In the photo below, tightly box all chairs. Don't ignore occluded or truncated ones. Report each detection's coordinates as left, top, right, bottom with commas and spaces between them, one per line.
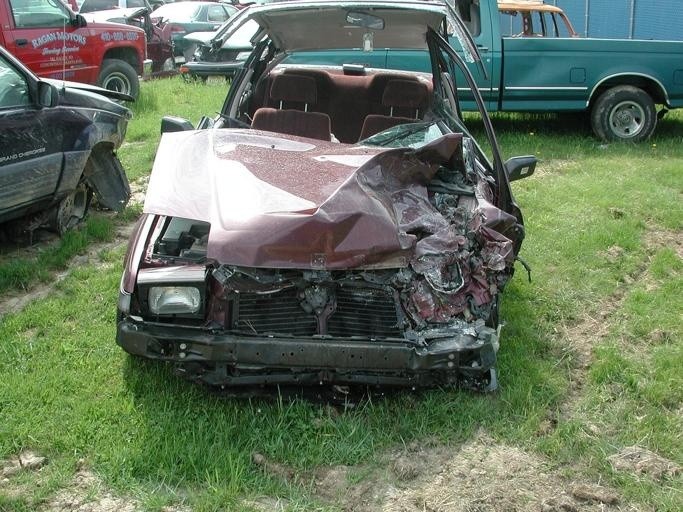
248, 62, 435, 143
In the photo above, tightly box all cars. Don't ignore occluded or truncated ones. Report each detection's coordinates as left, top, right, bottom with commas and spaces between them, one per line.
0, 43, 136, 244
79, 0, 683, 145
113, 2, 538, 398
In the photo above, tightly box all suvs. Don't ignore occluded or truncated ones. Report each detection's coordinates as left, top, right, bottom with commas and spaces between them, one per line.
0, 0, 147, 106
